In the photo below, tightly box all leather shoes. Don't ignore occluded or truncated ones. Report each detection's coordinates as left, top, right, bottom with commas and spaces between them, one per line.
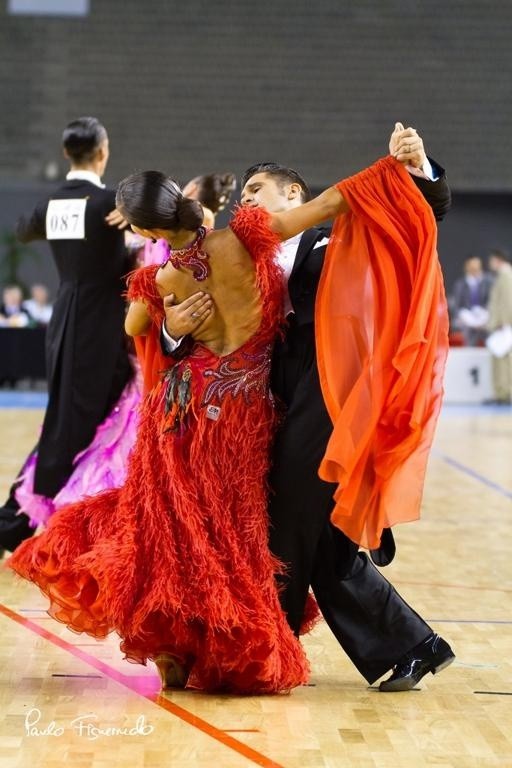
379, 633, 456, 692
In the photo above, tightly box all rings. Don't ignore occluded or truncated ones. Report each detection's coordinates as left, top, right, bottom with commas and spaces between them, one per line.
192, 312, 200, 319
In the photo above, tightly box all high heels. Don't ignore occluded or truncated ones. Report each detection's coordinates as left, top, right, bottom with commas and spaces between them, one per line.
154, 654, 189, 689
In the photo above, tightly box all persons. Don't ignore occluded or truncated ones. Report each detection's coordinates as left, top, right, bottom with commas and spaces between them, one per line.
23, 282, 53, 388
160, 122, 456, 692
446, 255, 494, 347
14, 173, 237, 529
0, 286, 32, 389
2, 126, 410, 695
0, 116, 157, 560
474, 249, 512, 405
